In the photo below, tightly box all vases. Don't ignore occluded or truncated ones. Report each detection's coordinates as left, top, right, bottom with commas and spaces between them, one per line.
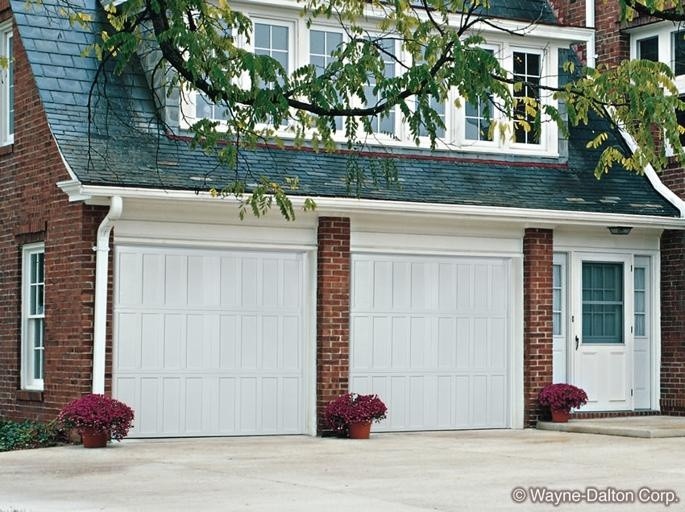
550, 406, 570, 422
348, 421, 371, 439
82, 428, 106, 448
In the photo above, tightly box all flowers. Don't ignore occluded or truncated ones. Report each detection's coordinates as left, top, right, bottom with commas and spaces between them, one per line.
538, 384, 586, 412
56, 393, 135, 441
324, 393, 387, 433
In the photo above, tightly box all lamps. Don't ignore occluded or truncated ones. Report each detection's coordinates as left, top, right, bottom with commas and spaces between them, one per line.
608, 227, 632, 234
